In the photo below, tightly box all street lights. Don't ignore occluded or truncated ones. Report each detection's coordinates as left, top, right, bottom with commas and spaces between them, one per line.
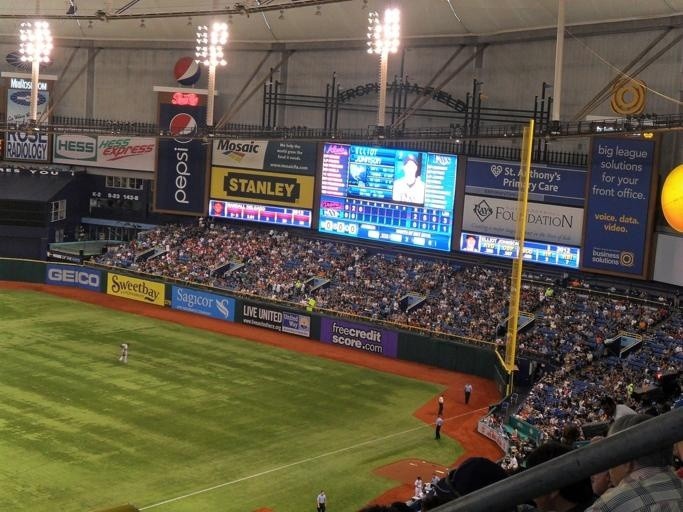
19, 20, 54, 127
365, 7, 403, 138
195, 22, 229, 127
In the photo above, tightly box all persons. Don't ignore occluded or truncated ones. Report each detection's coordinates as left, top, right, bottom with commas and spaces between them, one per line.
212, 201, 225, 216
392, 156, 425, 204
